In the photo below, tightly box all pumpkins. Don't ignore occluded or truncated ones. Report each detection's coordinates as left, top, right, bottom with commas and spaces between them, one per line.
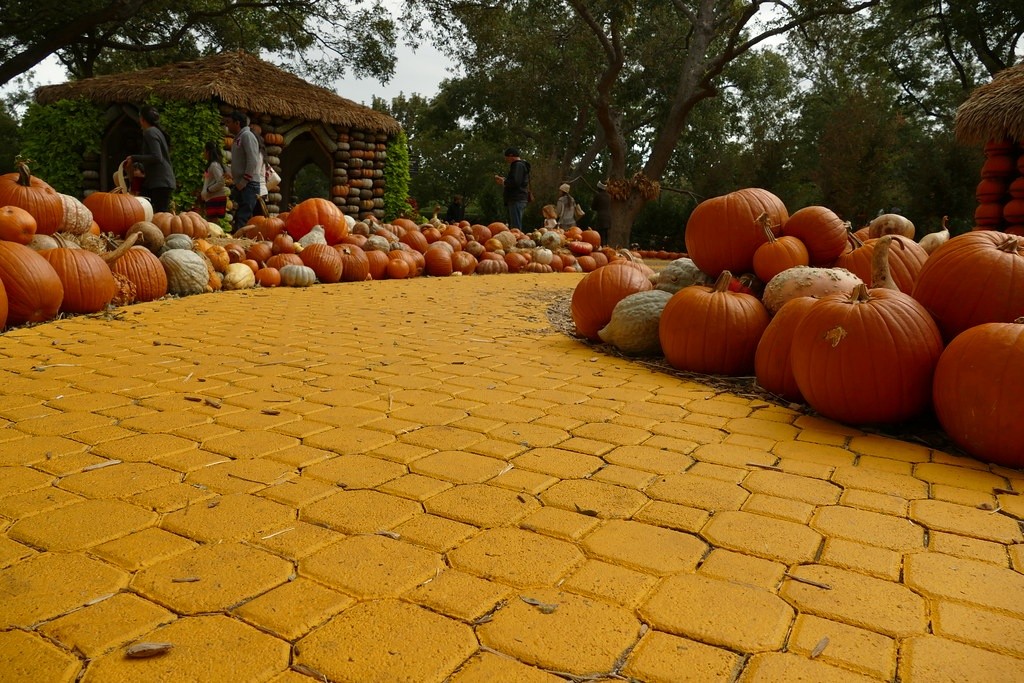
0, 105, 609, 332
569, 137, 1024, 469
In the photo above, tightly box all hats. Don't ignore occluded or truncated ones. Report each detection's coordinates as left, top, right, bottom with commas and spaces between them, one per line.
113, 160, 134, 196
504, 148, 520, 157
558, 183, 570, 194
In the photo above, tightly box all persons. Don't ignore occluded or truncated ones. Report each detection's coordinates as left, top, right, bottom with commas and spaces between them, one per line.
542, 205, 559, 230
446, 195, 461, 224
226, 111, 260, 235
494, 148, 532, 231
249, 125, 269, 218
199, 140, 226, 226
552, 184, 576, 230
125, 110, 176, 215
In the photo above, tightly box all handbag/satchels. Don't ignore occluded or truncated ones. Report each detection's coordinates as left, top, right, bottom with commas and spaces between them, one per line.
526, 191, 535, 205
574, 204, 585, 221
264, 160, 281, 192
